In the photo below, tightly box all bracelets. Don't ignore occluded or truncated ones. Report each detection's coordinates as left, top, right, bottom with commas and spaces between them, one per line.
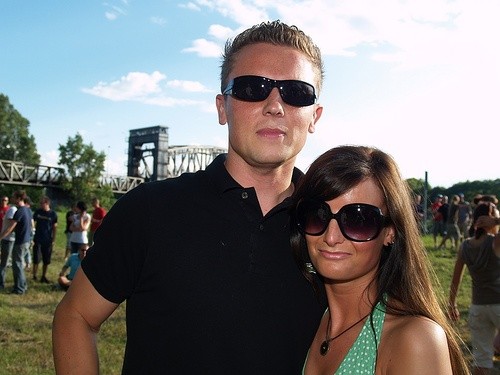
449, 304, 457, 309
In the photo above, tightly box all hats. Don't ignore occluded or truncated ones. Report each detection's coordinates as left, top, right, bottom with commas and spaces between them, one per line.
436, 194, 443, 198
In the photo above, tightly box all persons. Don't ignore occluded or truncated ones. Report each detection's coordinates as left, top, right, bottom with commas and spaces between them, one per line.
52, 23, 329, 375
0, 189, 105, 293
413, 193, 500, 375
285, 145, 472, 375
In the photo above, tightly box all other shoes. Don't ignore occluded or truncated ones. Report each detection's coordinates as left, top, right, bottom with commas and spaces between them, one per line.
32, 275, 37, 281
40, 276, 50, 283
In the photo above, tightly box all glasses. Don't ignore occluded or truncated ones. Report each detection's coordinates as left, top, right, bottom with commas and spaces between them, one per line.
223, 75, 317, 107
81, 248, 87, 251
293, 198, 391, 242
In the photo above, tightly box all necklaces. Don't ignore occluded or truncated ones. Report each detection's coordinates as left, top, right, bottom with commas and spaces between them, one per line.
320, 313, 370, 356
